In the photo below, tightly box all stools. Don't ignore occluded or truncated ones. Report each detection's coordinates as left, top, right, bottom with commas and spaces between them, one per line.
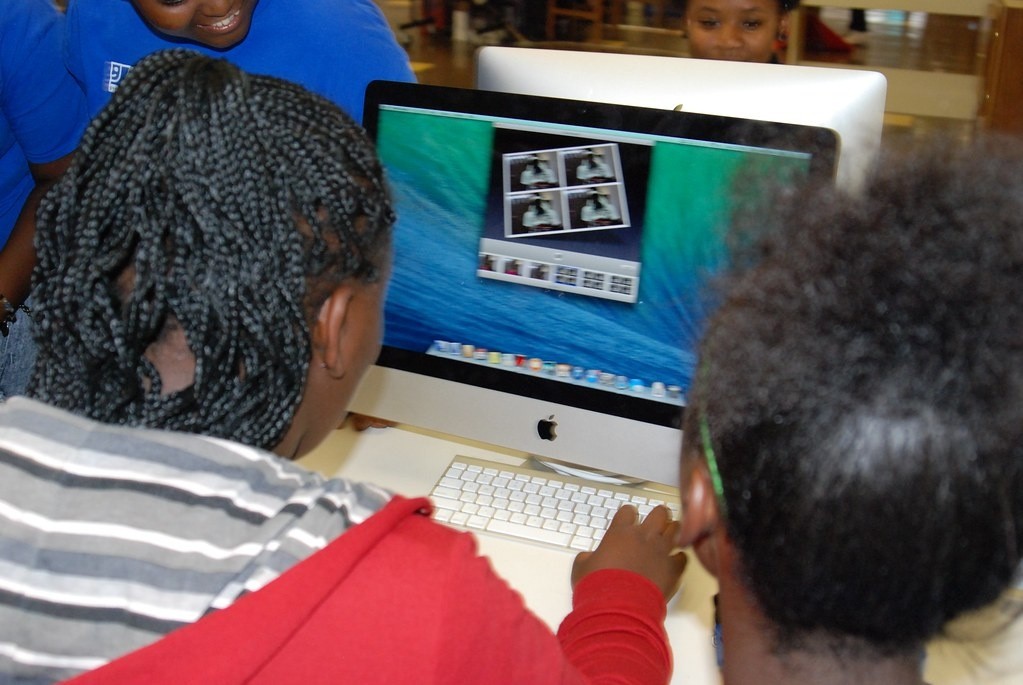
546, 0, 619, 43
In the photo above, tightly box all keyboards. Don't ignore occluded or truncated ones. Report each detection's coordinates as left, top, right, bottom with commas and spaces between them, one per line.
425, 455, 685, 552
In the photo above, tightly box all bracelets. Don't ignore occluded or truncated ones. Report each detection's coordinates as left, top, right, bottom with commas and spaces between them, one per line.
0, 293, 15, 315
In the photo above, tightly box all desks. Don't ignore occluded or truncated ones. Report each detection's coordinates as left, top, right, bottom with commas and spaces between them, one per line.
292, 417, 1023, 684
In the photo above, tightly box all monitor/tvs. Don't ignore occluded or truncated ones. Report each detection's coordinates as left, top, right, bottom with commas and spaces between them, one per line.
346, 46, 887, 492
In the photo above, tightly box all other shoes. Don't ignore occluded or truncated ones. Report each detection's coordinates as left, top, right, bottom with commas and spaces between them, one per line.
842, 29, 871, 47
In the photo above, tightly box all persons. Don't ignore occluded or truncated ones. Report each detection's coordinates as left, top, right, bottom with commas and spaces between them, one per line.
0, 0, 90, 337
0, 49, 688, 685
679, 145, 1023, 685
64, 0, 418, 132
681, 0, 793, 63
